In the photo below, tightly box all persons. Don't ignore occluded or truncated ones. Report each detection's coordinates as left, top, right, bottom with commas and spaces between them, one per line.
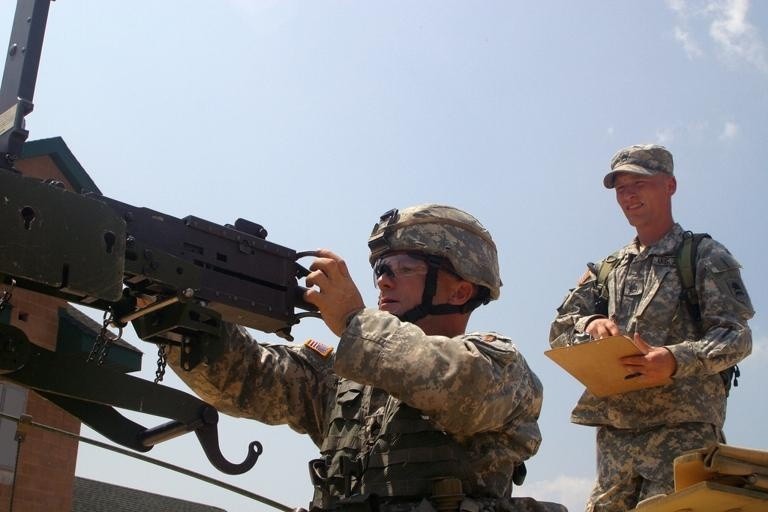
148, 202, 545, 511
546, 143, 756, 511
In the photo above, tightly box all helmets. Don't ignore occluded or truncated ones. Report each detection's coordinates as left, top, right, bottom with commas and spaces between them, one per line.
368, 202, 500, 301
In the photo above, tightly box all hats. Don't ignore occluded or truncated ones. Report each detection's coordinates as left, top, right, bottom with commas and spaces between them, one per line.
602, 144, 674, 189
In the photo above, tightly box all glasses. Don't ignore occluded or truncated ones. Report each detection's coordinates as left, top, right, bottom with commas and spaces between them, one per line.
372, 251, 428, 288
